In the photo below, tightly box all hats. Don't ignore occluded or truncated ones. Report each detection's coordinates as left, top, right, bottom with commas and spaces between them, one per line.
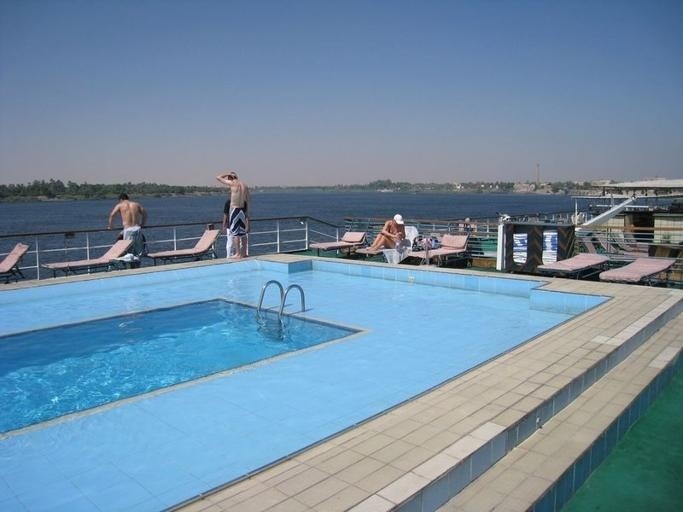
393, 214, 404, 226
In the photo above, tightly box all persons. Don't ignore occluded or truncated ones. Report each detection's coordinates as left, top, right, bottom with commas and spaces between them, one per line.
108, 193, 146, 268
365, 213, 405, 252
222, 198, 248, 257
216, 171, 249, 259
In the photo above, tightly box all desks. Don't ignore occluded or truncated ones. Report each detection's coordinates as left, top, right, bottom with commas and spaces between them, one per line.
110, 258, 140, 269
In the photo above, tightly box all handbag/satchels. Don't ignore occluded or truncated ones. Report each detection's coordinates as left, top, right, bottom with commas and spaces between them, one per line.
411, 235, 440, 253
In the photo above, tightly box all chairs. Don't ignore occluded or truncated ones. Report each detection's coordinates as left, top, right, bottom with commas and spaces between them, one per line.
309, 216, 472, 265
144, 229, 220, 266
40, 239, 135, 277
536, 253, 677, 286
0, 242, 30, 283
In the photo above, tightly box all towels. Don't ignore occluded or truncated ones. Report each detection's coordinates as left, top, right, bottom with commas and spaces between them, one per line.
121, 252, 135, 262
